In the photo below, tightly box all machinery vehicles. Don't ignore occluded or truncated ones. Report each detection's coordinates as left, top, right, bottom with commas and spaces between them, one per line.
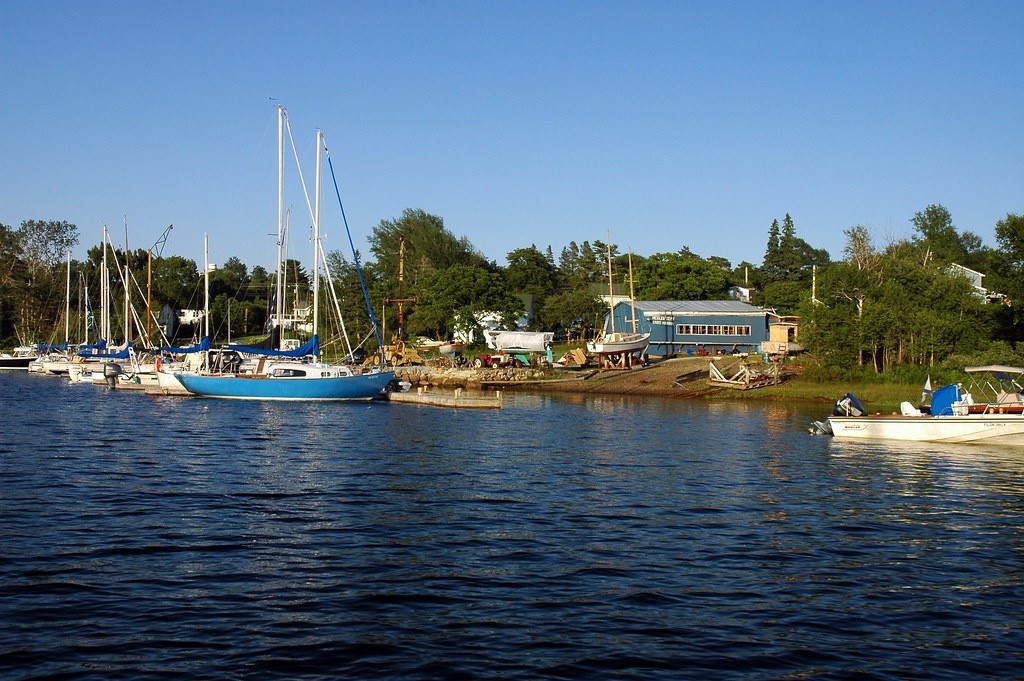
363, 234, 426, 366
474, 353, 521, 369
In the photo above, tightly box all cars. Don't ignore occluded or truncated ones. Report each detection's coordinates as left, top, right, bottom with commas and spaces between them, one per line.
345, 347, 367, 365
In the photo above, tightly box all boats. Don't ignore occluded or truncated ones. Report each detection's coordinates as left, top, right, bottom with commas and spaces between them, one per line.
808, 365, 1024, 440
438, 342, 463, 357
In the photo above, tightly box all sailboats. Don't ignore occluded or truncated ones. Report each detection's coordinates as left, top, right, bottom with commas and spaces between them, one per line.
0, 96, 396, 403
586, 229, 650, 355
